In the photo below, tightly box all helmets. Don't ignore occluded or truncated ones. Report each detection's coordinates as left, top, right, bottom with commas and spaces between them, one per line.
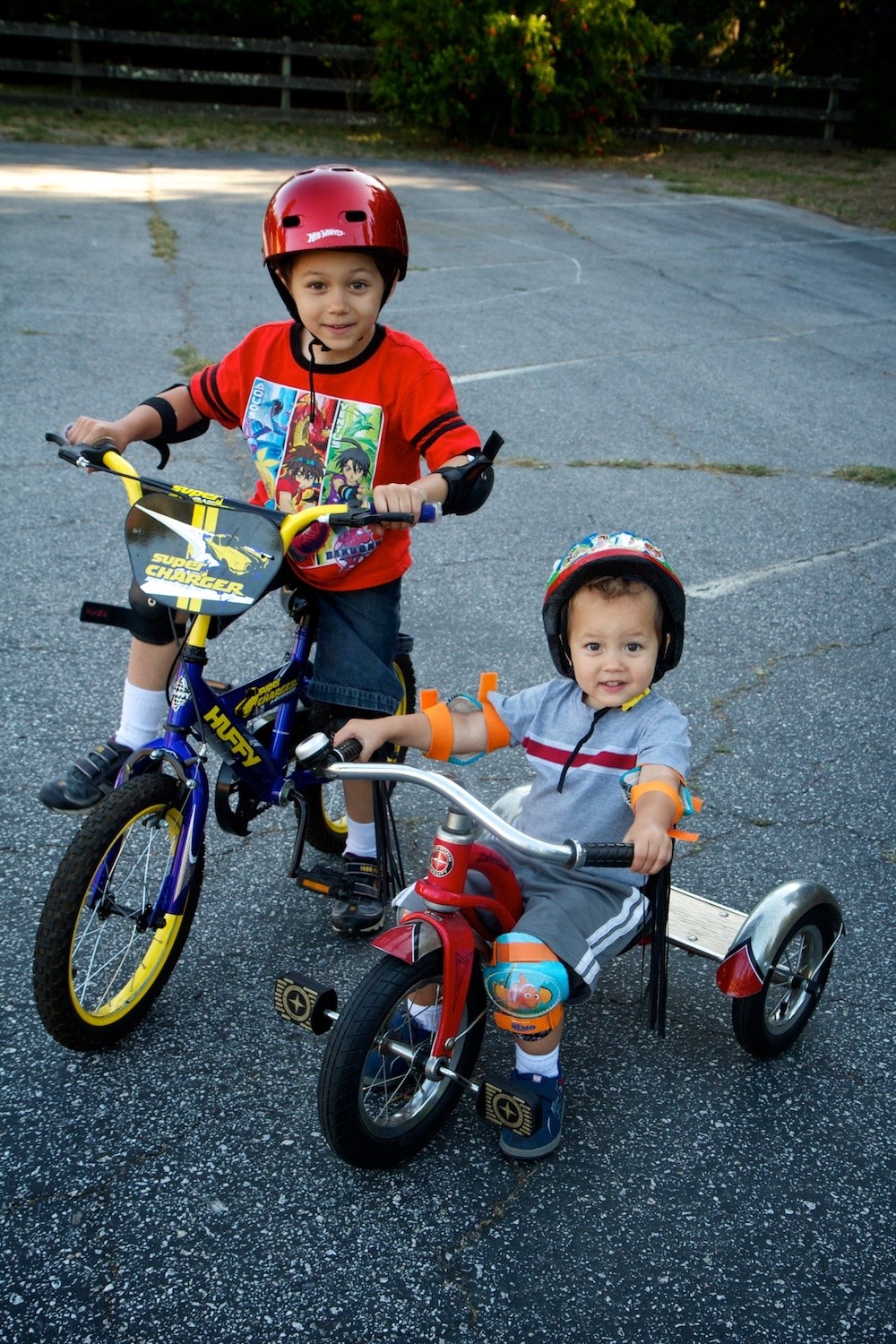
261, 163, 409, 285
540, 532, 686, 677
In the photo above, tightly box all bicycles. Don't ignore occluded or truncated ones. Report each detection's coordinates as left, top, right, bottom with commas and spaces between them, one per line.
32, 424, 438, 1053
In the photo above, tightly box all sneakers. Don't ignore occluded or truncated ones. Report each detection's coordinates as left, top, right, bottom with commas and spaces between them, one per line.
330, 851, 388, 936
500, 1067, 567, 1158
359, 1004, 437, 1088
38, 735, 133, 817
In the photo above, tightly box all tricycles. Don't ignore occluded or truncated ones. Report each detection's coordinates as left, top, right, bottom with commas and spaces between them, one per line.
290, 733, 849, 1166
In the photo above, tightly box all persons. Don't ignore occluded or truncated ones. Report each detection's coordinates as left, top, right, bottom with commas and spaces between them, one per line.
36, 161, 505, 935
333, 532, 703, 1156
